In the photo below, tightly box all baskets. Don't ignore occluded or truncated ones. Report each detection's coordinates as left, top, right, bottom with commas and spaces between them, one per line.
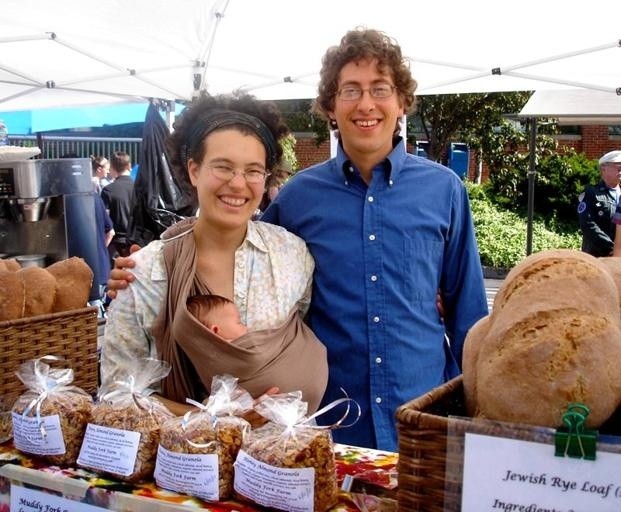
0, 304, 100, 426
394, 373, 621, 512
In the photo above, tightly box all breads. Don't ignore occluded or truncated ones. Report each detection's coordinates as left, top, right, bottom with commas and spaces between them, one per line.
0, 256, 94, 320
462, 248, 621, 430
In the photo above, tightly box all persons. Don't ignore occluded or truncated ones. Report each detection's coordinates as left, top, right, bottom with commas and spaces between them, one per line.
611, 196, 621, 257
97, 87, 449, 433
106, 27, 489, 448
578, 149, 621, 257
260, 161, 294, 212
102, 150, 138, 268
46, 152, 116, 304
184, 293, 249, 343
88, 154, 110, 192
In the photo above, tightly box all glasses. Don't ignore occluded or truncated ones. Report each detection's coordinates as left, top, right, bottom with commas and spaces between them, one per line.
196, 158, 273, 185
336, 83, 398, 101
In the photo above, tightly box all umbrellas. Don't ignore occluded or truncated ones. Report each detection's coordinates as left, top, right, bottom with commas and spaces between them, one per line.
124, 102, 197, 247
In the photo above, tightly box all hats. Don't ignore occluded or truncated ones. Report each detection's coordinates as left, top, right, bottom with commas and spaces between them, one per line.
276, 162, 293, 174
599, 150, 621, 166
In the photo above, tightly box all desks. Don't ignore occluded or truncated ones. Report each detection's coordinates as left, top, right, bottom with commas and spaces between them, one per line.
0, 437, 399, 512
